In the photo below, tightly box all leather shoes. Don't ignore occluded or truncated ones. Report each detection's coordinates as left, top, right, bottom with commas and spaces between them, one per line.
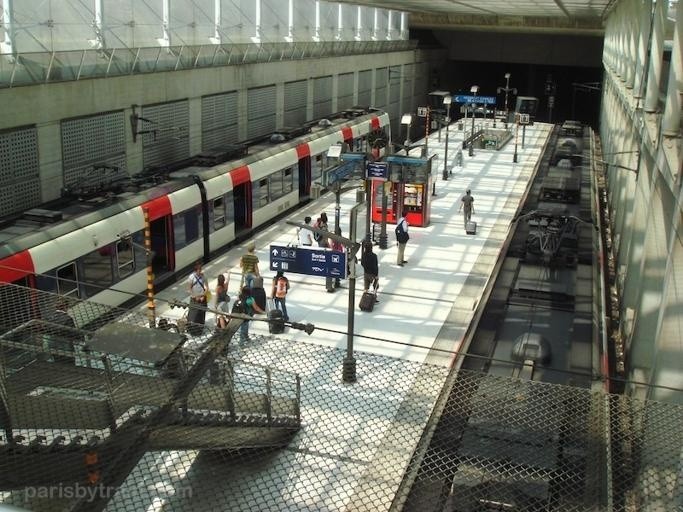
401, 261, 407, 263
398, 264, 404, 267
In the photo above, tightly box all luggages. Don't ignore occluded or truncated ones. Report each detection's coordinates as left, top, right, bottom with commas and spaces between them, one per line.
267, 309, 284, 334
465, 219, 477, 236
186, 304, 205, 337
359, 291, 377, 313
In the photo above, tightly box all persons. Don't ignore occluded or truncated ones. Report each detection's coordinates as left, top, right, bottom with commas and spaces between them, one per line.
360, 242, 380, 304
186, 262, 209, 298
237, 287, 266, 347
239, 243, 260, 288
312, 212, 326, 237
214, 271, 231, 320
297, 216, 312, 246
270, 269, 290, 321
216, 294, 230, 329
331, 227, 344, 253
396, 210, 409, 267
44, 297, 82, 367
314, 216, 330, 248
458, 189, 474, 231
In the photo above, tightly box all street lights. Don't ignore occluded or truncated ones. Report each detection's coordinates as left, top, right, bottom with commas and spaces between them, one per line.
369, 113, 430, 159
455, 84, 488, 156
307, 139, 367, 286
429, 95, 462, 180
116, 227, 156, 329
493, 73, 517, 123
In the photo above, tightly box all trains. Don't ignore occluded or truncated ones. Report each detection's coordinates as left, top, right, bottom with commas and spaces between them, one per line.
0, 102, 389, 357
390, 119, 617, 512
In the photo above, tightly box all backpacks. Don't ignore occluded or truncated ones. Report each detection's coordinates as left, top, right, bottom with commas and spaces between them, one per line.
313, 221, 325, 242
274, 276, 288, 299
395, 219, 410, 244
230, 298, 249, 322
